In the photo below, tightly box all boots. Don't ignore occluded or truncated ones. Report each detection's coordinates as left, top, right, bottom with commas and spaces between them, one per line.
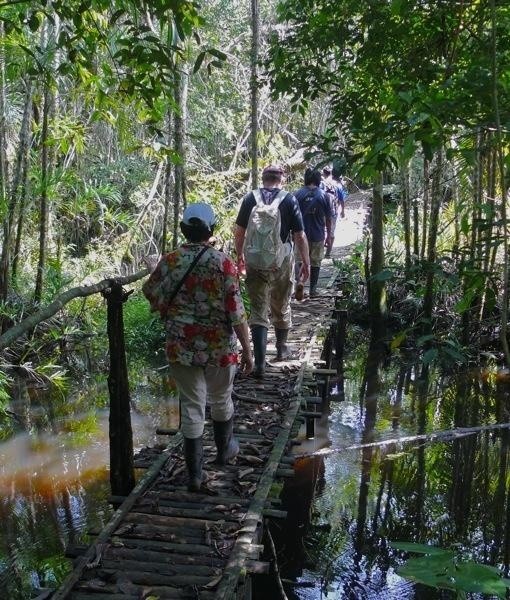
296, 262, 304, 301
309, 267, 320, 297
184, 436, 208, 490
214, 415, 239, 467
325, 237, 334, 258
250, 326, 267, 375
274, 328, 292, 358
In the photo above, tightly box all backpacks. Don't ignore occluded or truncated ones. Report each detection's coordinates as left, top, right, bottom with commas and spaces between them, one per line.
241, 188, 292, 271
321, 183, 338, 217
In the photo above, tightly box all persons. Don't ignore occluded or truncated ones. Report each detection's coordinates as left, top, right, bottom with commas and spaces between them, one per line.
141, 202, 256, 492
321, 165, 345, 259
234, 164, 312, 377
291, 167, 333, 301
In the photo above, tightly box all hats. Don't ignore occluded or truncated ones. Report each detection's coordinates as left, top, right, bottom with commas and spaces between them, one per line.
262, 164, 284, 178
182, 203, 216, 227
